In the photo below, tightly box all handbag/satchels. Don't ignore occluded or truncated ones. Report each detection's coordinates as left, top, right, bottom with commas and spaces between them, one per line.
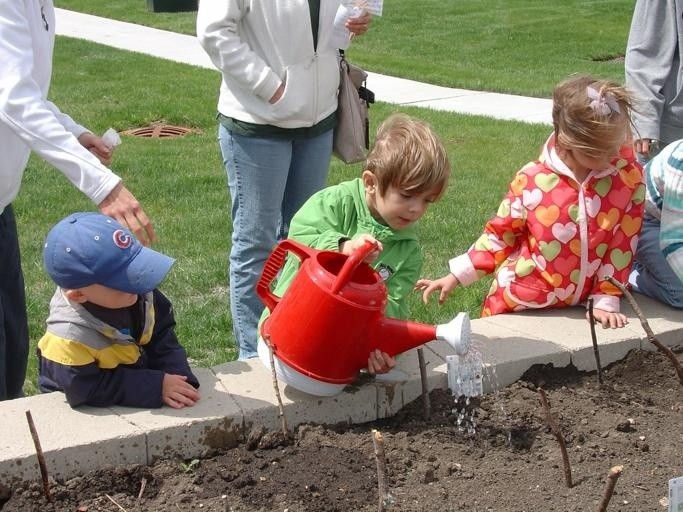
332, 59, 371, 165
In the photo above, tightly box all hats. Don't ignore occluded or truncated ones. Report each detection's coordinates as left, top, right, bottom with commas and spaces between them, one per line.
44, 212, 178, 295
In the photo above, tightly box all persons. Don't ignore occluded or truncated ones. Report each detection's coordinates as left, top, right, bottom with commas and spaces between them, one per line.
195, 1, 371, 360
622, 1, 683, 168
0, 0, 156, 401
625, 138, 681, 310
255, 110, 451, 376
35, 211, 201, 410
411, 72, 646, 331
